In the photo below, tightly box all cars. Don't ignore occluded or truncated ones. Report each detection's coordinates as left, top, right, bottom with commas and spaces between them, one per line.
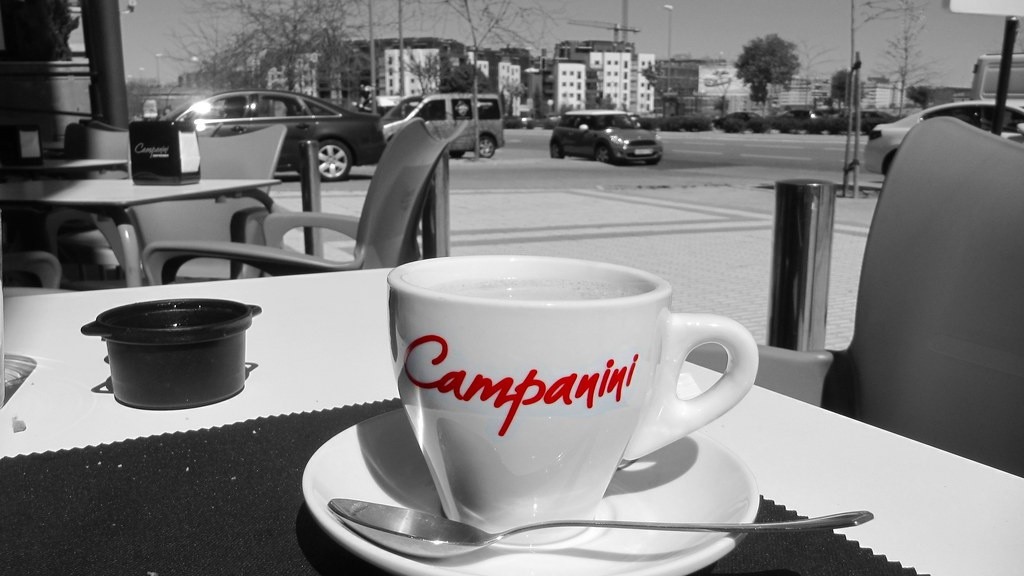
548, 108, 665, 167
772, 107, 829, 136
714, 112, 767, 134
858, 99, 1024, 175
834, 108, 906, 136
159, 89, 385, 181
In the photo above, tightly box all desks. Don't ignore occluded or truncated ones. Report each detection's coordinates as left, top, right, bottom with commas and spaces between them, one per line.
0, 158, 128, 253
0, 179, 283, 288
0, 266, 1024, 576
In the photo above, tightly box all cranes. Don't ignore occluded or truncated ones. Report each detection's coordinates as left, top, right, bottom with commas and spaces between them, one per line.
564, 16, 643, 45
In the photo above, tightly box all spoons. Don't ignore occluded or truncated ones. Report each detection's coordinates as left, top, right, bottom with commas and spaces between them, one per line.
328, 498, 874, 558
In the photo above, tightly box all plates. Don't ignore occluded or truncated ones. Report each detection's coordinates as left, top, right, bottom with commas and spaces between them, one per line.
302, 407, 753, 576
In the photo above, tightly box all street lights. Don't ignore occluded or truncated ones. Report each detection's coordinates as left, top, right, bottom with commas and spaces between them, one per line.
664, 4, 673, 94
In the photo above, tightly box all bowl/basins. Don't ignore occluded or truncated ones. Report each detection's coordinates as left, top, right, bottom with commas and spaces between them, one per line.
80, 298, 262, 410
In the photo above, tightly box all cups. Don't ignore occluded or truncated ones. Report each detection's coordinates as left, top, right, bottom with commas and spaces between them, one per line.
385, 255, 759, 545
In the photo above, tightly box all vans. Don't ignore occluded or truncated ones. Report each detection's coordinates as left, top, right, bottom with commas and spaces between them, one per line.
971, 53, 1024, 104
382, 92, 506, 159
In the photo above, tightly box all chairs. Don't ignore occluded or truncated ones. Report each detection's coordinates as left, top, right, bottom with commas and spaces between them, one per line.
141, 117, 469, 288
686, 117, 1024, 477
37, 122, 130, 256
127, 123, 291, 285
